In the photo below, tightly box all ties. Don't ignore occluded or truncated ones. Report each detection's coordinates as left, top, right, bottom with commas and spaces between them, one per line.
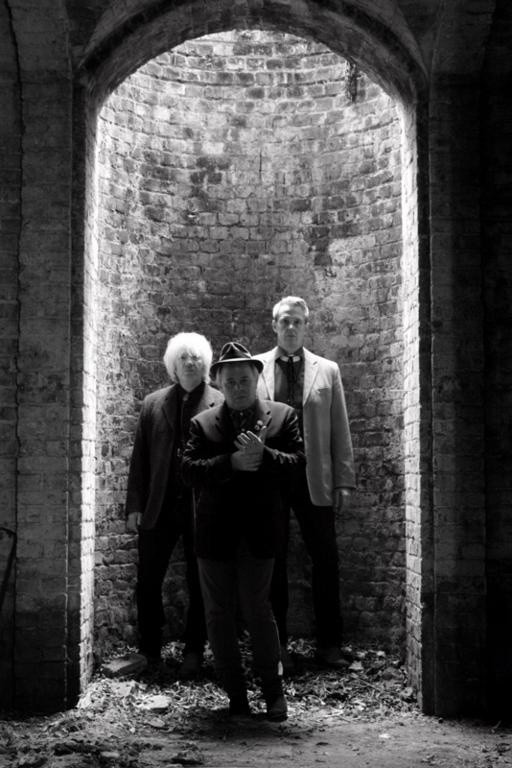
287, 356, 295, 406
181, 395, 193, 444
238, 411, 246, 434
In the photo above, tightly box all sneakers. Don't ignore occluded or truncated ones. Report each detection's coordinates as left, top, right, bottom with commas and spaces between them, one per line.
136, 661, 161, 687
178, 652, 204, 680
319, 647, 350, 666
280, 644, 295, 668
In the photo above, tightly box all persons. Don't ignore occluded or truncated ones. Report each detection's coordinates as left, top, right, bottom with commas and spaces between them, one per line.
177, 341, 307, 721
125, 331, 227, 674
254, 296, 357, 670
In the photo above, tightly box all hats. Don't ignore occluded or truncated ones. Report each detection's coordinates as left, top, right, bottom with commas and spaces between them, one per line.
210, 342, 263, 379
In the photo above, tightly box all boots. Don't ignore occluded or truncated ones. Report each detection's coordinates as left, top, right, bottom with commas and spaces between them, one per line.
221, 667, 252, 720
260, 672, 287, 719
